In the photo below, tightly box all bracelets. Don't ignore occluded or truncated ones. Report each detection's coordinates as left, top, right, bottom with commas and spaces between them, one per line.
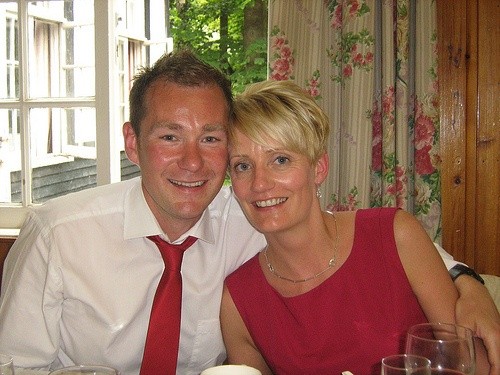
446, 264, 486, 287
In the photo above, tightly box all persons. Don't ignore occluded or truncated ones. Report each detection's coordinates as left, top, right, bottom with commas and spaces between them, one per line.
0, 50, 499, 373
218, 80, 494, 375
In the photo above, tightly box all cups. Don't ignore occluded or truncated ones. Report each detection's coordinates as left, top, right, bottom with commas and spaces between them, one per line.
404, 322, 476, 375
381, 354, 432, 375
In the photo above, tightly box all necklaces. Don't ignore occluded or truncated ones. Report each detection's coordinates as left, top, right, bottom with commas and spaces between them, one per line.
264, 209, 336, 282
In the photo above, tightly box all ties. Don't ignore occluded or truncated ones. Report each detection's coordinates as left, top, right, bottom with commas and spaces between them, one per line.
138, 235, 198, 375
